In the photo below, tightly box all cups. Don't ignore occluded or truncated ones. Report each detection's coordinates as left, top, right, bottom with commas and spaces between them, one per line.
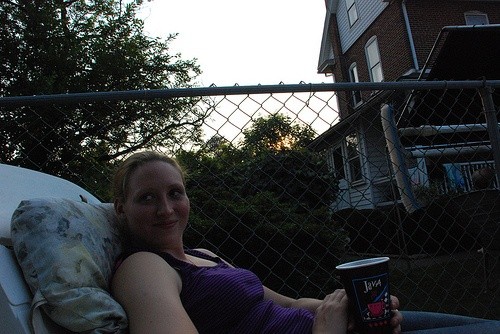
336, 256, 394, 334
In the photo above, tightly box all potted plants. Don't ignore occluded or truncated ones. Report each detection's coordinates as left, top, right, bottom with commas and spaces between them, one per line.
413, 182, 434, 211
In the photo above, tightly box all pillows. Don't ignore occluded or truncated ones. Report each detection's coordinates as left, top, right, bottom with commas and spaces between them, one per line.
11, 196, 128, 334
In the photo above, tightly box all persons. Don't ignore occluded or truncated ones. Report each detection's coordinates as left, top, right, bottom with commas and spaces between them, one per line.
471, 168, 495, 191
111, 151, 500, 334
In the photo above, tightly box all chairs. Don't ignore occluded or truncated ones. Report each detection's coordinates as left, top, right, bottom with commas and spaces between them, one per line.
0, 163, 106, 333
